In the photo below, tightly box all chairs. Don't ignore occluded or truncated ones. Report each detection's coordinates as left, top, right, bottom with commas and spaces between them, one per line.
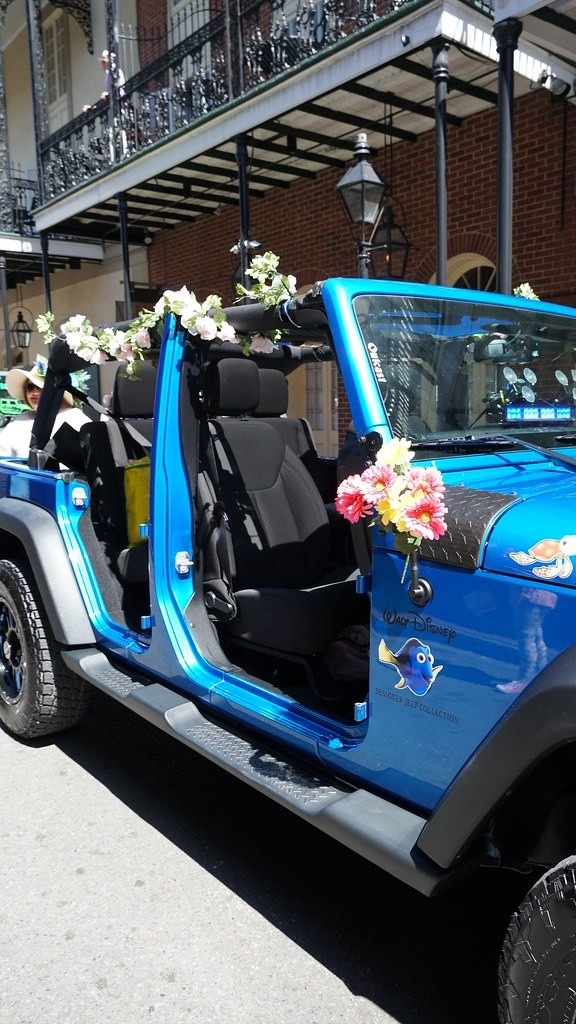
106, 352, 369, 691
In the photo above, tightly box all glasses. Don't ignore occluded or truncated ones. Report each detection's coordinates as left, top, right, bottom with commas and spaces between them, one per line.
27, 383, 40, 391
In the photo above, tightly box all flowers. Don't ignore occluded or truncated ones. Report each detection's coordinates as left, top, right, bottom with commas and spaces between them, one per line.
511, 281, 539, 301
330, 434, 451, 584
232, 252, 303, 329
34, 284, 288, 396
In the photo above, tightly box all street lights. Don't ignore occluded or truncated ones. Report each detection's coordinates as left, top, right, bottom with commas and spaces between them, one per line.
332, 132, 386, 279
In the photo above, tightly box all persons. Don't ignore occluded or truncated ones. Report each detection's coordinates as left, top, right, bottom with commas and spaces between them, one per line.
83, 49, 125, 160
496, 587, 558, 695
0, 355, 93, 459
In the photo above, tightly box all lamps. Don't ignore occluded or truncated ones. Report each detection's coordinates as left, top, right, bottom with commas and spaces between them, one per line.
8, 280, 32, 347
366, 97, 410, 284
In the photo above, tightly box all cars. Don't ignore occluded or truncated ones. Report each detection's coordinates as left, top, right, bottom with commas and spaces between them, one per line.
3, 275, 576, 1023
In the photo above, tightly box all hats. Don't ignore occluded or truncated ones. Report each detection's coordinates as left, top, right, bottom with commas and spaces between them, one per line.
5, 353, 73, 408
98, 51, 110, 62
